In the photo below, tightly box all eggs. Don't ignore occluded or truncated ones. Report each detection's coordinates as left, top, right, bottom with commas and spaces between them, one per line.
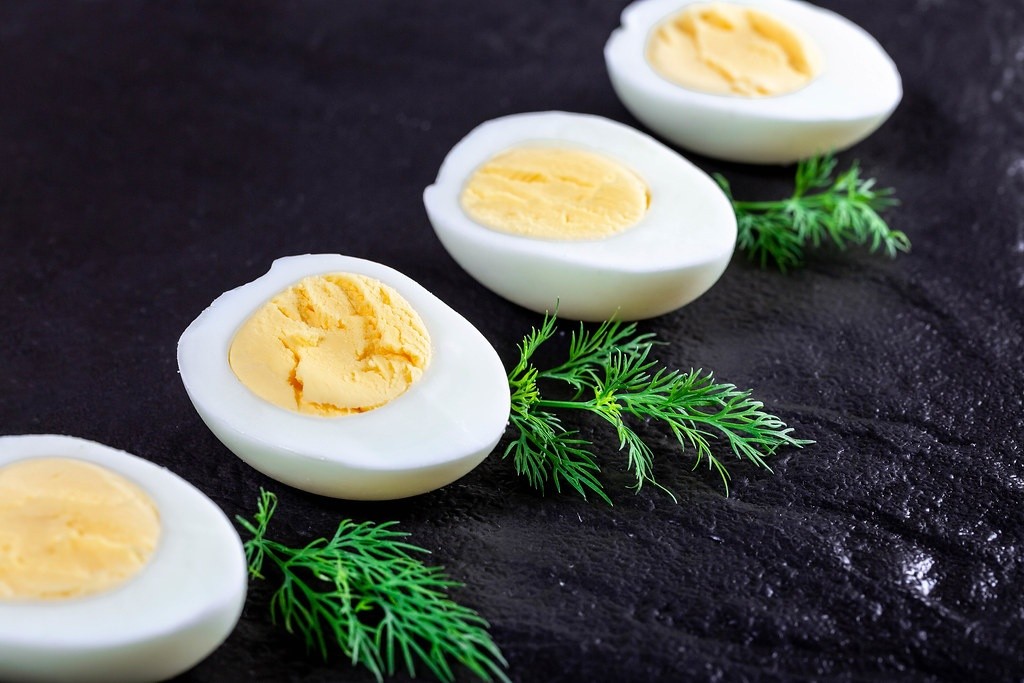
1, 0, 905, 680
